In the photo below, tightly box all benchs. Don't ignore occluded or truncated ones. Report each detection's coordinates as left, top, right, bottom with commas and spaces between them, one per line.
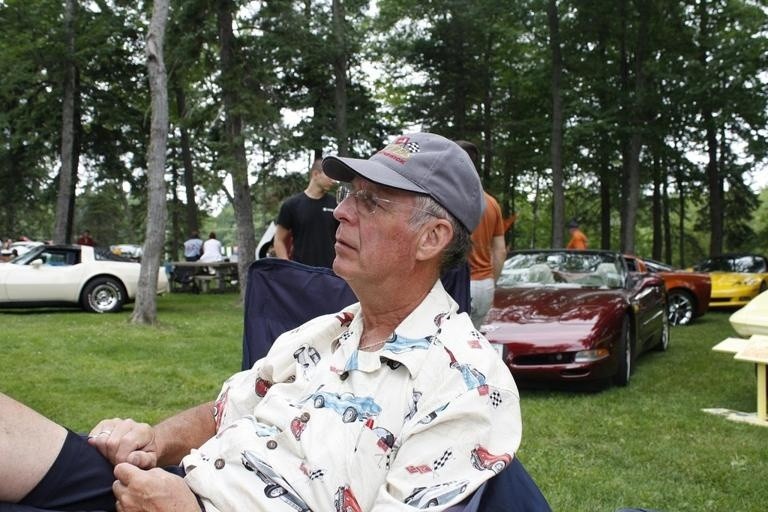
164, 259, 239, 294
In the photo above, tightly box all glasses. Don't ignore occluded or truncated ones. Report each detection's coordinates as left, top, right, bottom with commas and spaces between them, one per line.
335, 185, 439, 218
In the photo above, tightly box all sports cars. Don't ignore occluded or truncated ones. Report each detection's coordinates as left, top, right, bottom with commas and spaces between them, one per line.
622, 252, 768, 326
473, 249, 672, 393
0, 242, 170, 313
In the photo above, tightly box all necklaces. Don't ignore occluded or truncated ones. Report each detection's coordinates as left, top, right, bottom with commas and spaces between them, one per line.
359, 341, 385, 349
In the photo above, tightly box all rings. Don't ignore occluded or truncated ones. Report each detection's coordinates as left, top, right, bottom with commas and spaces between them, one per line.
87, 436, 97, 439
99, 431, 111, 436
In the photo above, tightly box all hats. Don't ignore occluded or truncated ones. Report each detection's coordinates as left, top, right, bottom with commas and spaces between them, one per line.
320, 132, 484, 235
568, 221, 577, 228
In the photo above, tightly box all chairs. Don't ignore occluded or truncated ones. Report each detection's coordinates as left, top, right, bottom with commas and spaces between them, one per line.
240, 256, 552, 512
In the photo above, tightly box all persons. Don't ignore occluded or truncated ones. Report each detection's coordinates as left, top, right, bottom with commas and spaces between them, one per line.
77, 230, 96, 246
0, 131, 522, 512
183, 231, 204, 262
454, 139, 506, 332
200, 232, 224, 274
275, 159, 339, 270
254, 220, 277, 260
566, 221, 588, 250
